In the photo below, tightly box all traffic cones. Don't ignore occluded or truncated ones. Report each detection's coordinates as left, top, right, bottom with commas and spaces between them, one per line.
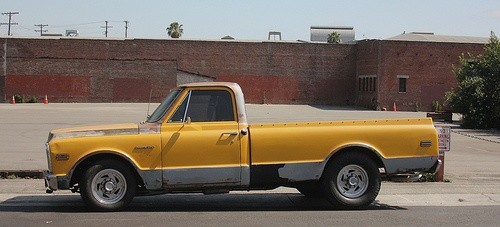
44, 92, 48, 104
392, 100, 397, 111
382, 107, 387, 111
11, 95, 15, 104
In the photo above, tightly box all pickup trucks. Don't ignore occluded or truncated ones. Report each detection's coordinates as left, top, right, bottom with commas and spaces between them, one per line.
41, 81, 439, 210
